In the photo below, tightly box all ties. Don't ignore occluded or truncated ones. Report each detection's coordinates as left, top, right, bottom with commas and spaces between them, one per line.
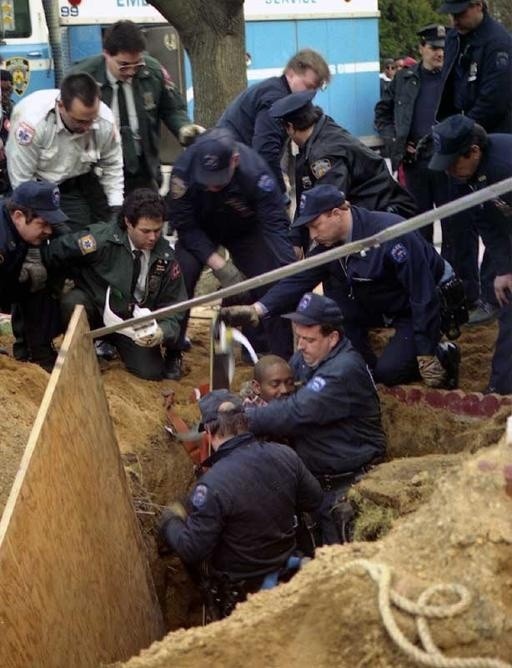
131, 250, 142, 293
115, 80, 139, 174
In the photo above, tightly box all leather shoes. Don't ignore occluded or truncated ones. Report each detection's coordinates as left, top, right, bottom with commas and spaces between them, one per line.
163, 350, 181, 379
94, 340, 118, 360
468, 301, 499, 325
446, 343, 461, 389
34, 349, 58, 372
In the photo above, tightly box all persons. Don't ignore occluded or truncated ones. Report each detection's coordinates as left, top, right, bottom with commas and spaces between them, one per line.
1, 2, 511, 396
158, 291, 388, 616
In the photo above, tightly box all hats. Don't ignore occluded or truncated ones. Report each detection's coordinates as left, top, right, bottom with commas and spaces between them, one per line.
190, 129, 235, 186
416, 26, 451, 50
13, 180, 71, 225
292, 184, 346, 230
428, 115, 474, 172
270, 90, 313, 117
439, 0, 475, 14
280, 292, 345, 327
198, 389, 245, 433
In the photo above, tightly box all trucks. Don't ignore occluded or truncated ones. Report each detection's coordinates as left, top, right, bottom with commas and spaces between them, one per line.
0, 1, 385, 193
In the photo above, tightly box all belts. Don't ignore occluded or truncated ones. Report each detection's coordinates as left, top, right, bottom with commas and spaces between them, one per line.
315, 457, 384, 493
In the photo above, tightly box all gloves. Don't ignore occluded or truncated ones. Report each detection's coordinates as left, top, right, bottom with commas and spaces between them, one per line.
419, 355, 445, 388
134, 318, 163, 348
19, 249, 48, 293
180, 124, 205, 145
212, 259, 250, 303
214, 306, 259, 342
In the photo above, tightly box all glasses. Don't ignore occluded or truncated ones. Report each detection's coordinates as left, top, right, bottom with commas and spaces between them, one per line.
109, 54, 146, 72
